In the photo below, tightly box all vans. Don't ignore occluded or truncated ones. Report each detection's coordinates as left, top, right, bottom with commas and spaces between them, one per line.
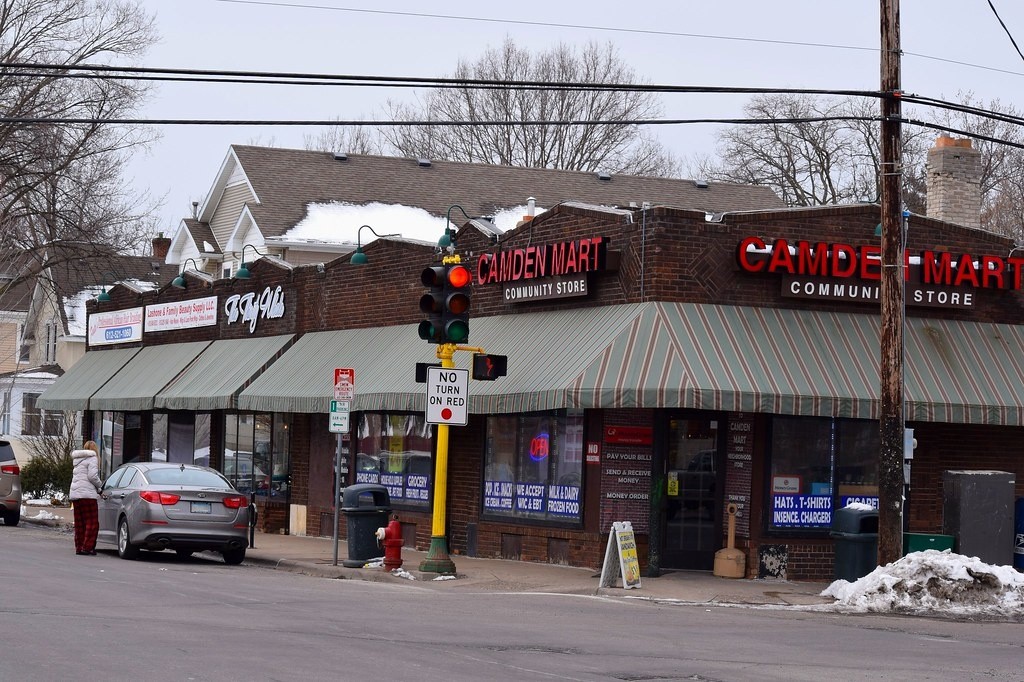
0, 441, 22, 527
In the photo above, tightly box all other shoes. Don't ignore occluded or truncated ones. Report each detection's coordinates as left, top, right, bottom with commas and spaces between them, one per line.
82, 547, 98, 556
75, 549, 86, 555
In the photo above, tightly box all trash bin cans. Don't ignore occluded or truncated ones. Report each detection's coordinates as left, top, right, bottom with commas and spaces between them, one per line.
341, 484, 394, 568
829, 508, 879, 583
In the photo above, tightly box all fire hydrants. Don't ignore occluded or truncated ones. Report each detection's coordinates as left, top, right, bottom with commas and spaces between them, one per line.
375, 514, 404, 572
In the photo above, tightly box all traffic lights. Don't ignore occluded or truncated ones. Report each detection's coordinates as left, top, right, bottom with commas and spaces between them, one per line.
442, 263, 470, 344
473, 353, 508, 381
418, 264, 443, 344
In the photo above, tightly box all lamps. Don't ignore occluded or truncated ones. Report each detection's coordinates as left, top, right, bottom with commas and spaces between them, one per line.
350, 225, 401, 265
437, 205, 482, 248
874, 223, 882, 238
171, 259, 214, 290
96, 272, 121, 302
234, 244, 282, 280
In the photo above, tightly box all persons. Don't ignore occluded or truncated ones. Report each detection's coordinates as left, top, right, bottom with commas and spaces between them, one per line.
69, 440, 109, 556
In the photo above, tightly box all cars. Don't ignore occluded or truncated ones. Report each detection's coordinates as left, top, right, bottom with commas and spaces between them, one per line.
97, 462, 250, 564
195, 457, 270, 497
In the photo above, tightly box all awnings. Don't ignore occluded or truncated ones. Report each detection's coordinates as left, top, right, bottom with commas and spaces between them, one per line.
238, 302, 1024, 428
90, 340, 214, 410
35, 346, 142, 412
154, 333, 295, 409
